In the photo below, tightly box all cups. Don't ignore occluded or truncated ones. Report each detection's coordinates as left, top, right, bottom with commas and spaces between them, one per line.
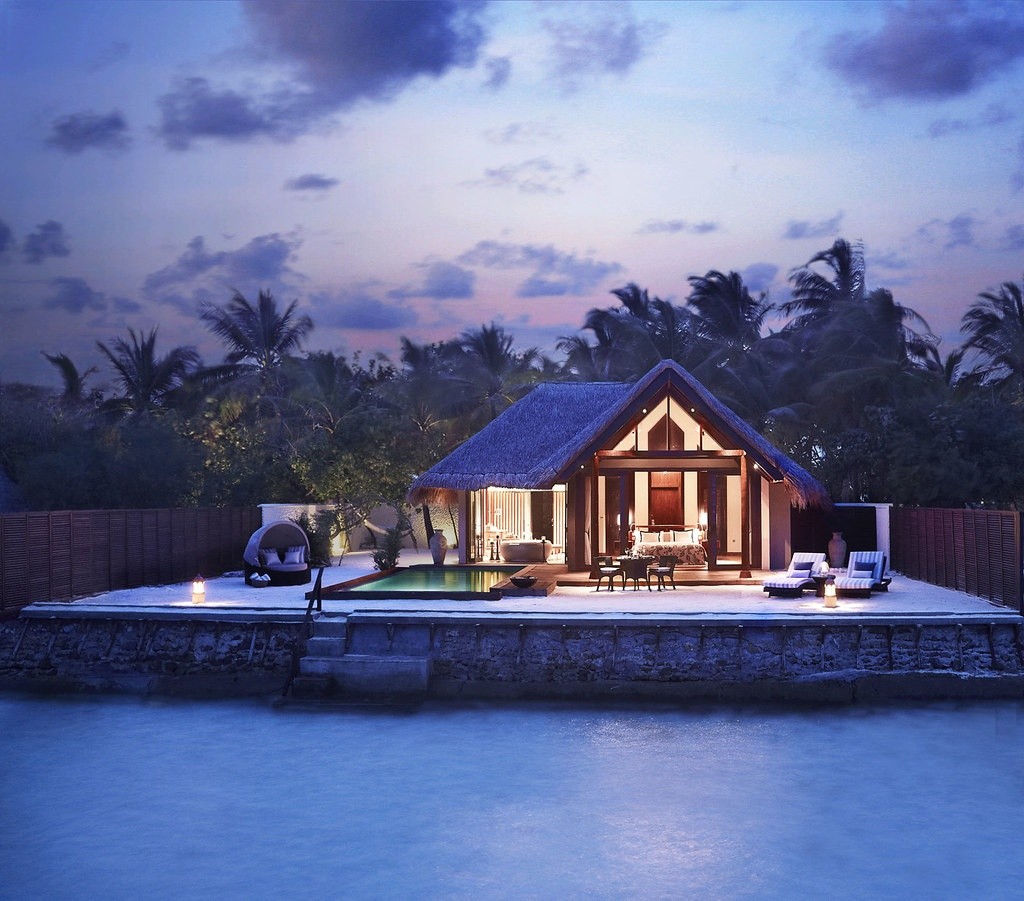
625, 547, 629, 556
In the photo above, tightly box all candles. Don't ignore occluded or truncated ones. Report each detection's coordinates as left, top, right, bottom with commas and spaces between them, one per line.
542, 536, 545, 542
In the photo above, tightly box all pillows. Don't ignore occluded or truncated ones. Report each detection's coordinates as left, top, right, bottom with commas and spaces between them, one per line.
672, 529, 695, 545
640, 531, 660, 544
259, 548, 282, 566
789, 561, 814, 578
283, 545, 305, 564
851, 562, 876, 579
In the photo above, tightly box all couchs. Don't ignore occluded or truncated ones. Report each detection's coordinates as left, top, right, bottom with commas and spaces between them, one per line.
242, 519, 312, 588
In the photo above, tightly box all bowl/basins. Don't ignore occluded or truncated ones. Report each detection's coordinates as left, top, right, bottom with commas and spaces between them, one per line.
510, 576, 538, 588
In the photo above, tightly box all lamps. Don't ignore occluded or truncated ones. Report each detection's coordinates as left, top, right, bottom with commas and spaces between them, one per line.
823, 578, 838, 609
192, 573, 206, 604
820, 561, 829, 575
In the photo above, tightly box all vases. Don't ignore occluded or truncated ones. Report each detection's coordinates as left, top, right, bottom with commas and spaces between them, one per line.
650, 519, 656, 525
429, 529, 447, 566
827, 531, 847, 569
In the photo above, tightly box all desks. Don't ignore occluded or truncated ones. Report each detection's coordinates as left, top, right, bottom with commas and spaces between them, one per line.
486, 530, 505, 557
811, 574, 836, 597
615, 555, 655, 593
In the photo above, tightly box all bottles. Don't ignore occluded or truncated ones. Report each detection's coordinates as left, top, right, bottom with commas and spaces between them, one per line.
829, 531, 846, 568
630, 543, 642, 558
429, 529, 447, 564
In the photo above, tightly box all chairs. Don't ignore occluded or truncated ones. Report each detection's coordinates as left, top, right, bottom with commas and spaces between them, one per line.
593, 556, 625, 592
648, 556, 678, 592
762, 551, 892, 599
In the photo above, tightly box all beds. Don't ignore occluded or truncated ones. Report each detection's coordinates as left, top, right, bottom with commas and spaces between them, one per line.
632, 525, 707, 568
